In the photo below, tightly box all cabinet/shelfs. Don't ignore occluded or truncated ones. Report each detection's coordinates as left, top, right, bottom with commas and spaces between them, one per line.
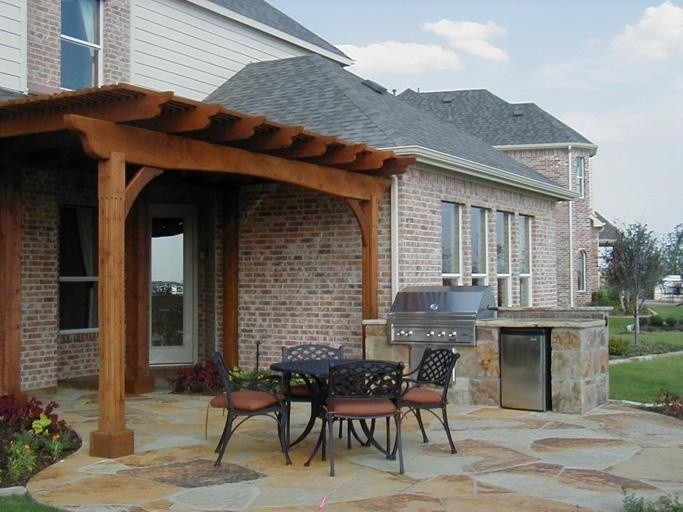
497, 328, 552, 412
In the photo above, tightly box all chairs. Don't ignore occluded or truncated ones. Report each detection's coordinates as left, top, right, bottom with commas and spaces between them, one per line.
278, 342, 352, 456
320, 356, 407, 480
362, 345, 464, 459
211, 348, 297, 468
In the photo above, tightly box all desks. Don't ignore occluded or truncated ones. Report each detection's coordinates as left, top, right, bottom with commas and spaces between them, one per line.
267, 355, 411, 469
